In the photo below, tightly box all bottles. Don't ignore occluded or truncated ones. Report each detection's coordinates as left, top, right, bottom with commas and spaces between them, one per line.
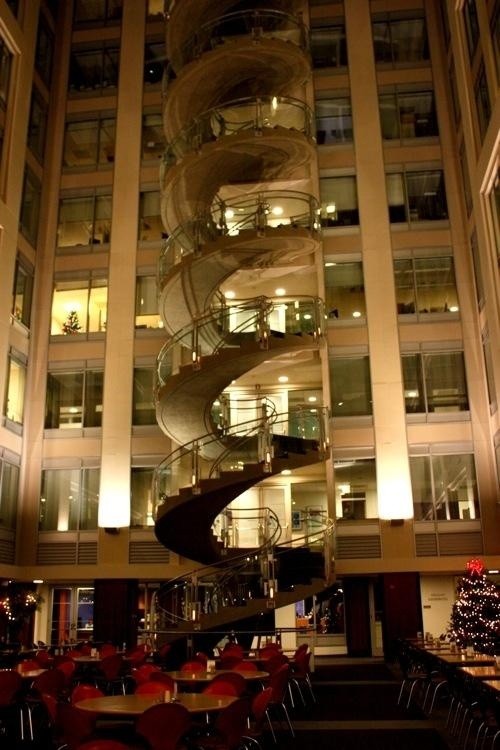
160, 690, 172, 703
90, 648, 96, 658
1, 633, 10, 647
415, 632, 500, 669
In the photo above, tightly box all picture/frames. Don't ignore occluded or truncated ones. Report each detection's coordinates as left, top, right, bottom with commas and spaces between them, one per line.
291, 510, 303, 531
306, 505, 323, 527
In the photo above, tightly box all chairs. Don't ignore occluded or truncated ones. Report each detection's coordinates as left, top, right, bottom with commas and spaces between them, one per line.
395, 634, 500, 750
